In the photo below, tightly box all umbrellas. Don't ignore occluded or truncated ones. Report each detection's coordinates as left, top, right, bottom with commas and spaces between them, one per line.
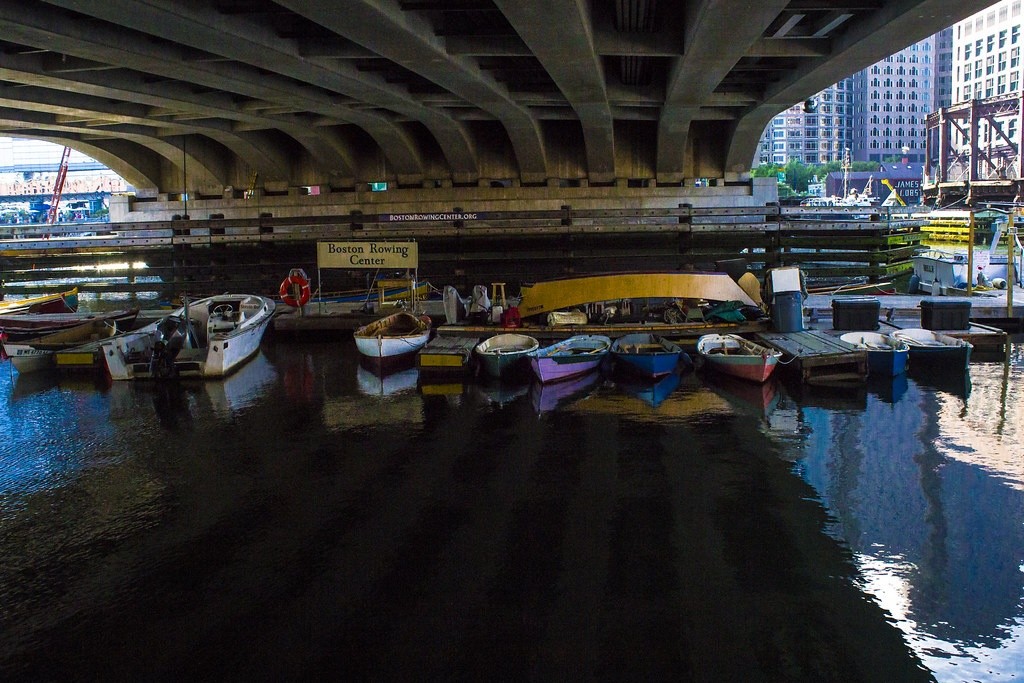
57, 207, 92, 213
0, 209, 40, 217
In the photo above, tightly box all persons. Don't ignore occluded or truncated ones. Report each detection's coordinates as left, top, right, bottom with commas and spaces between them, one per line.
77, 213, 81, 218
14, 214, 18, 224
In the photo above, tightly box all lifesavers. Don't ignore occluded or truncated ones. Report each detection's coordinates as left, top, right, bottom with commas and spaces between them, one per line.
279, 275, 311, 306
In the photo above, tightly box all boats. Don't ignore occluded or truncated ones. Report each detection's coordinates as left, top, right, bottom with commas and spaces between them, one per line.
0, 283, 140, 380
102, 291, 278, 389
352, 308, 432, 357
839, 328, 910, 377
610, 329, 684, 380
694, 331, 784, 383
476, 331, 540, 376
889, 323, 974, 368
523, 331, 610, 386
